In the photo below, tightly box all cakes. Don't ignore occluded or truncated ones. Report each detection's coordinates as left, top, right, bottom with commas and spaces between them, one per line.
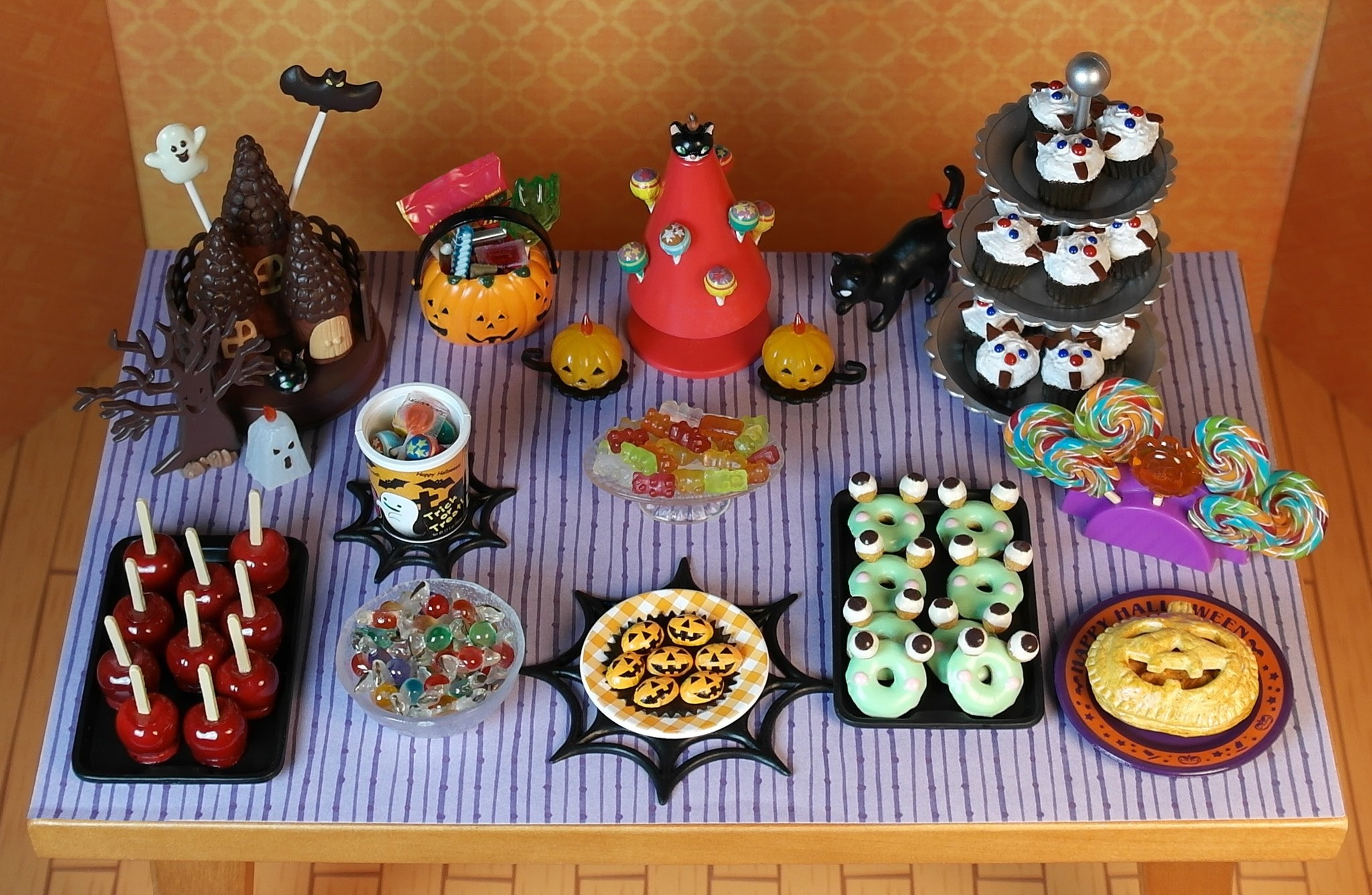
71, 64, 381, 481
1083, 600, 1260, 737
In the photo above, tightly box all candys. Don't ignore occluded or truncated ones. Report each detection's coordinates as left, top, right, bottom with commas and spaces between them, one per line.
349, 585, 514, 717
629, 167, 661, 213
659, 221, 690, 265
703, 265, 738, 307
93, 527, 290, 768
371, 397, 458, 460
713, 143, 734, 180
728, 198, 776, 246
1002, 376, 1330, 560
591, 400, 779, 499
617, 240, 647, 279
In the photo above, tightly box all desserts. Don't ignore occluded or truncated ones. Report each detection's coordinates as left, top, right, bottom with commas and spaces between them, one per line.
846, 471, 1039, 717
970, 192, 1160, 310
1021, 79, 1163, 212
605, 613, 743, 708
958, 292, 1141, 405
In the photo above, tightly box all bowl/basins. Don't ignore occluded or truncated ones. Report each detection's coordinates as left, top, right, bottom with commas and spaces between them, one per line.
334, 575, 524, 736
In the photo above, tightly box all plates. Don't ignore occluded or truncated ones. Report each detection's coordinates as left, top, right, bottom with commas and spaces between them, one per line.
572, 580, 771, 740
70, 529, 317, 789
829, 479, 1049, 733
1054, 592, 1293, 778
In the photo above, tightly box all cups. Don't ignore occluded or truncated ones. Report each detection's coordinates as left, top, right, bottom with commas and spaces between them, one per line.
354, 387, 478, 544
